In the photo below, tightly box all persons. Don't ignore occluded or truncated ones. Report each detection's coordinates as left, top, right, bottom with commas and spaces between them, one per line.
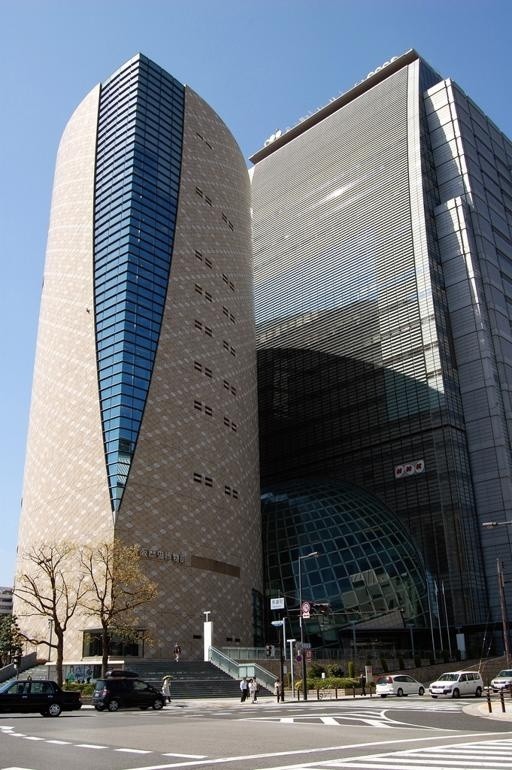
76, 667, 84, 681
162, 677, 172, 702
274, 679, 282, 695
265, 643, 271, 658
359, 673, 366, 696
17, 685, 24, 693
252, 677, 258, 703
248, 679, 255, 703
239, 677, 248, 702
68, 665, 75, 681
173, 641, 181, 663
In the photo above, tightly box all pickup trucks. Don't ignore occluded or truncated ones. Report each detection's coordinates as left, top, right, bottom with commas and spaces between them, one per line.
0, 680, 82, 717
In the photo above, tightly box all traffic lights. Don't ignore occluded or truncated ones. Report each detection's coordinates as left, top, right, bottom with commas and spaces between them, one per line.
12, 649, 22, 671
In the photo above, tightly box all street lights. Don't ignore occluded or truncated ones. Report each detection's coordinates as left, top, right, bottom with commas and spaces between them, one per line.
482, 521, 512, 528
271, 551, 317, 697
406, 623, 415, 656
203, 611, 211, 622
47, 617, 54, 680
348, 620, 360, 665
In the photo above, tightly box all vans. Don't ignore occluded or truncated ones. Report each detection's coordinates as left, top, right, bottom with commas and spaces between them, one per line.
429, 671, 484, 698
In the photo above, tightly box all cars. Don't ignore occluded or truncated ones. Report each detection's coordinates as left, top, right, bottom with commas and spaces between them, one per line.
376, 675, 425, 698
91, 679, 166, 712
491, 669, 512, 692
